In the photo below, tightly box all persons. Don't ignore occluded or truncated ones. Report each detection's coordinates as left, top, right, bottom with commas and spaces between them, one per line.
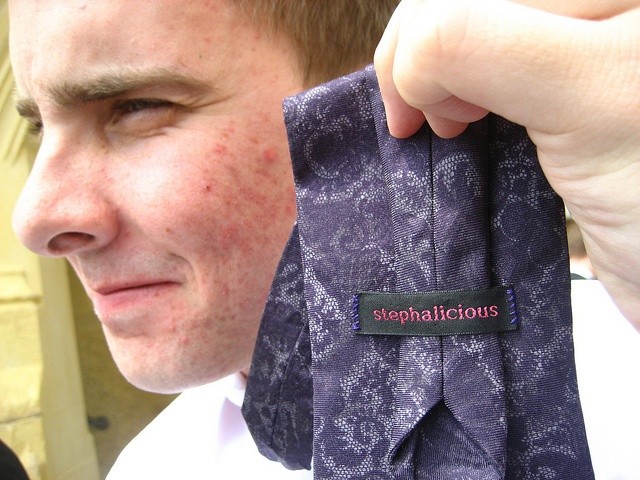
9, 1, 638, 480
